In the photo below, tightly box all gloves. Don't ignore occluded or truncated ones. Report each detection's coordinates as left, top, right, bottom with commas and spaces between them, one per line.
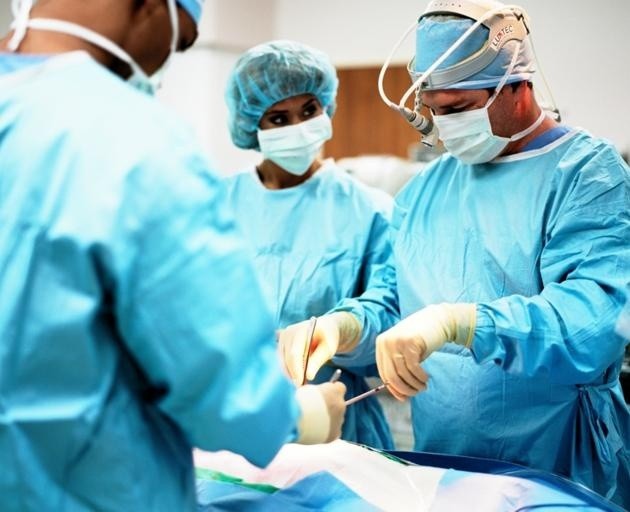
279, 311, 359, 387
297, 381, 346, 445
375, 301, 476, 401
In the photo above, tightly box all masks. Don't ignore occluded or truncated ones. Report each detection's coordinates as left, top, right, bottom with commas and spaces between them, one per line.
103, 0, 178, 94
255, 107, 331, 176
430, 80, 542, 162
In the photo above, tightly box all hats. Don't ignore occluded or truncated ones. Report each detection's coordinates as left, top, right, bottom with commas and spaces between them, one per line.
414, 15, 534, 90
226, 42, 338, 151
177, 0, 203, 51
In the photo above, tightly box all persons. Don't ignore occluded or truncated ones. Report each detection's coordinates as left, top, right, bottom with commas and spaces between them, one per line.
214, 39, 402, 452
277, 0, 630, 512
1, 1, 348, 512
